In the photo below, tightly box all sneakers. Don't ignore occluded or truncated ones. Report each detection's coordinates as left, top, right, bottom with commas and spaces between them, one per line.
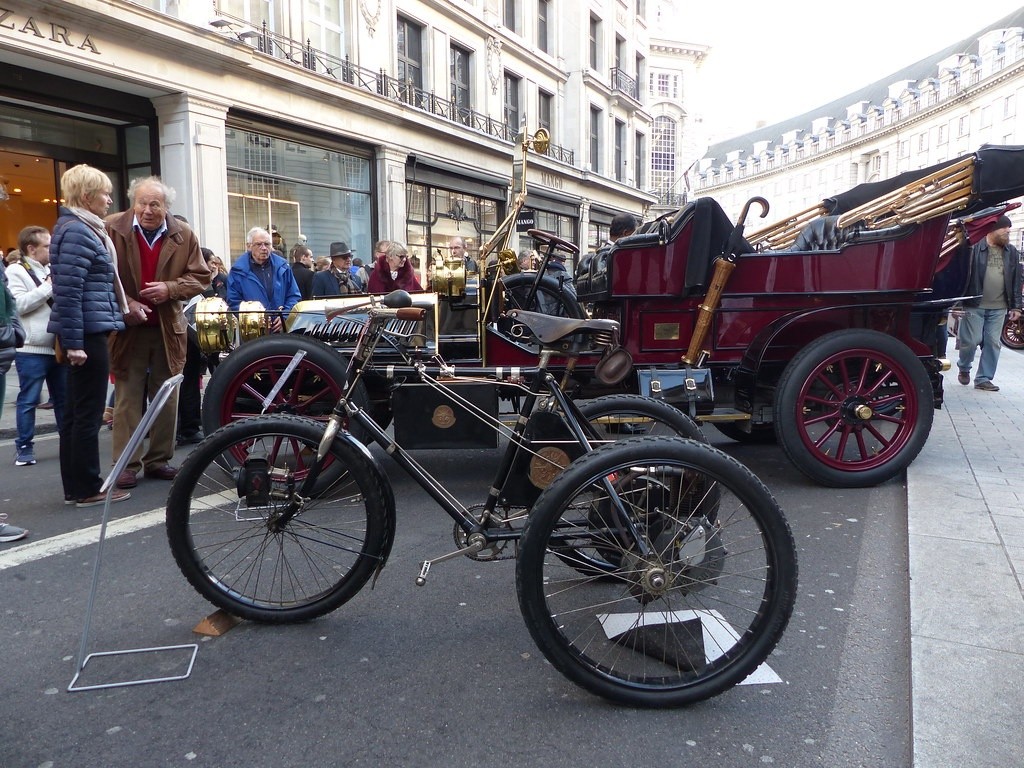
0, 513, 30, 542
12, 445, 37, 466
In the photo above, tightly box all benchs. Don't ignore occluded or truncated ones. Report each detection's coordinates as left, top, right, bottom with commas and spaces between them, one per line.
783, 210, 953, 287
577, 200, 697, 301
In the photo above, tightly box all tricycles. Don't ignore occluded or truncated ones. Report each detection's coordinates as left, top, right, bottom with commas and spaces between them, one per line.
166, 303, 798, 710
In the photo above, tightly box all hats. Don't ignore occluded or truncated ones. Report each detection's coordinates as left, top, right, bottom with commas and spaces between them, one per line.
327, 241, 356, 257
989, 215, 1012, 231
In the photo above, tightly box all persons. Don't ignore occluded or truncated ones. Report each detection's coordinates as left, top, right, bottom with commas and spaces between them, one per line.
48, 164, 131, 507
292, 241, 423, 301
104, 175, 301, 489
605, 213, 638, 246
518, 250, 535, 270
450, 236, 478, 271
266, 224, 287, 260
937, 215, 1022, 391
289, 235, 307, 262
0, 225, 63, 541
549, 251, 566, 271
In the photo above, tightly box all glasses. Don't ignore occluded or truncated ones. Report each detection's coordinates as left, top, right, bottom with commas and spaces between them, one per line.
336, 254, 353, 260
303, 239, 308, 243
396, 254, 405, 260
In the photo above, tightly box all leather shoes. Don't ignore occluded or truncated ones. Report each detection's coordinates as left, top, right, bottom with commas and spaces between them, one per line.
116, 471, 137, 489
180, 433, 205, 444
37, 402, 54, 409
144, 465, 177, 481
65, 478, 132, 507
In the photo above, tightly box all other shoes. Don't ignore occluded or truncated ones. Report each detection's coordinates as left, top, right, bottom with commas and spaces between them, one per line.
958, 368, 970, 385
974, 381, 1000, 391
103, 406, 114, 420
